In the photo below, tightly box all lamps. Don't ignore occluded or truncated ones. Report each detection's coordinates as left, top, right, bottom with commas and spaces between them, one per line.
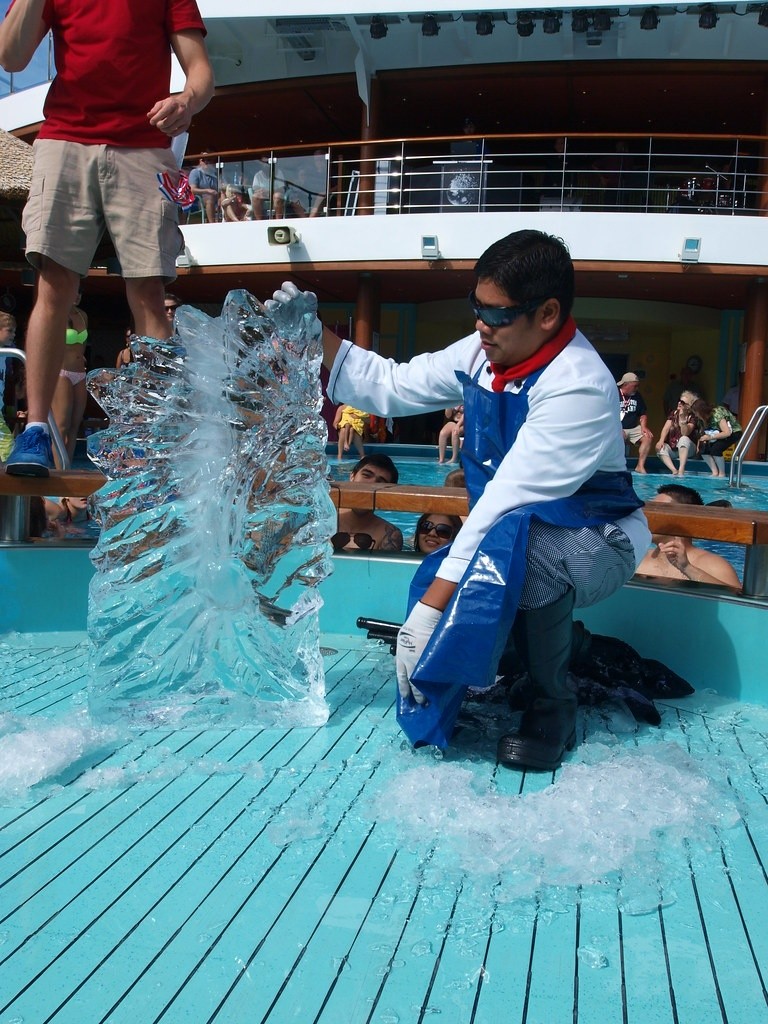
678, 237, 701, 261
369, 13, 388, 39
697, 3, 720, 29
513, 11, 536, 37
640, 6, 661, 30
758, 3, 768, 27
420, 235, 441, 260
543, 9, 563, 33
176, 241, 191, 267
422, 10, 441, 37
475, 12, 495, 35
571, 8, 614, 32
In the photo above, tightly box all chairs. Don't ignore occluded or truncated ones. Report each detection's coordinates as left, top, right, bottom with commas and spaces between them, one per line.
186, 195, 218, 224
247, 186, 292, 219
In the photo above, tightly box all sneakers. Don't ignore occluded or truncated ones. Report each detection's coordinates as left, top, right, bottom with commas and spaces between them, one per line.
3, 426, 50, 478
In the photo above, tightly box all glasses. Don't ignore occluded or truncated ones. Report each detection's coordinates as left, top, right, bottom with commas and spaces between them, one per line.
467, 287, 547, 326
679, 399, 688, 406
417, 520, 454, 538
332, 531, 377, 551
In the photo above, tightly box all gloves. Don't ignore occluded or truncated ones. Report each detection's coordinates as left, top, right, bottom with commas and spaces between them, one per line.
396, 600, 446, 705
265, 280, 323, 344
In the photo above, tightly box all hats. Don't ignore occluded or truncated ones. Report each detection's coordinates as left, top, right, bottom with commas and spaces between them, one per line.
616, 372, 639, 386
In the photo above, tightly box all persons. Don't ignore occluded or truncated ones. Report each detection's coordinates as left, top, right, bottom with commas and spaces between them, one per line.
334, 404, 386, 460
49, 288, 88, 470
444, 470, 468, 523
262, 229, 651, 772
453, 118, 491, 160
45, 495, 92, 525
655, 370, 743, 478
115, 328, 131, 367
335, 453, 403, 551
414, 512, 463, 554
0, 0, 214, 474
539, 139, 630, 210
634, 485, 741, 588
189, 148, 338, 217
714, 164, 752, 208
164, 293, 180, 322
0, 312, 28, 462
438, 406, 463, 463
617, 373, 653, 474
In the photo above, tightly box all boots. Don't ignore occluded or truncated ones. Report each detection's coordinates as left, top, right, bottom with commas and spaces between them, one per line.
499, 587, 575, 770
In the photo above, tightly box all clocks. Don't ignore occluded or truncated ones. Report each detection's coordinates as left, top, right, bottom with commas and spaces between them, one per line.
687, 355, 703, 374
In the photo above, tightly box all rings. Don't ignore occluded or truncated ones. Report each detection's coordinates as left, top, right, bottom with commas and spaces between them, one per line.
174, 124, 178, 130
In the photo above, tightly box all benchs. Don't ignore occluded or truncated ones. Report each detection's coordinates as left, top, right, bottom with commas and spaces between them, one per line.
0, 465, 768, 597
